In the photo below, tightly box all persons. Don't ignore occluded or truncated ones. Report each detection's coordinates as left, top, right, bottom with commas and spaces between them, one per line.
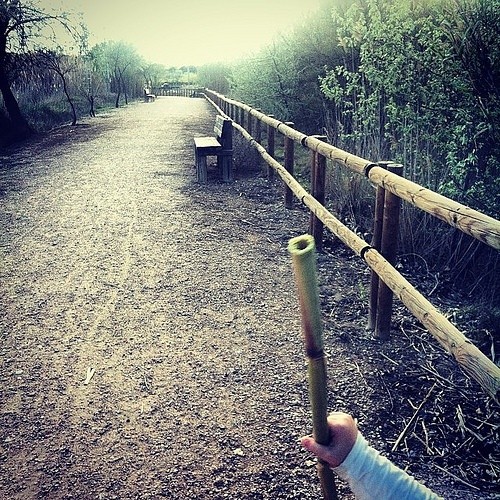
301, 412, 443, 500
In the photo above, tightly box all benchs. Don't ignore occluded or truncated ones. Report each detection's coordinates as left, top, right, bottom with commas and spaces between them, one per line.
193, 114, 234, 182
144, 89, 154, 102
